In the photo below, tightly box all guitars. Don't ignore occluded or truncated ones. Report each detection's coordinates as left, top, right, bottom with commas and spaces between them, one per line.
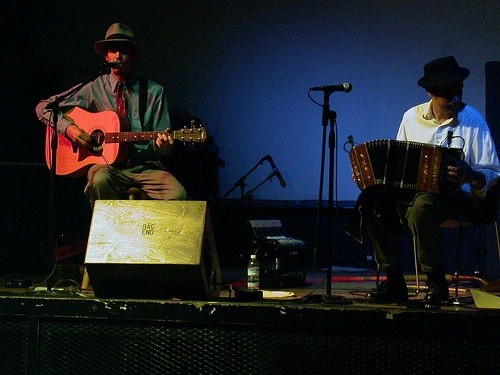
45, 105, 208, 176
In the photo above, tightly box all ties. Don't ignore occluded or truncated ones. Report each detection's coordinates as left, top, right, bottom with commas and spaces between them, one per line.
115, 81, 131, 168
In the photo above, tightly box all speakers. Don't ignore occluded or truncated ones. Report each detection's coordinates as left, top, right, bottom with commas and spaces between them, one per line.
84, 200, 223, 302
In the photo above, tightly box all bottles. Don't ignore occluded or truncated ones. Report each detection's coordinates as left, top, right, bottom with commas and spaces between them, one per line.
247, 254, 260, 290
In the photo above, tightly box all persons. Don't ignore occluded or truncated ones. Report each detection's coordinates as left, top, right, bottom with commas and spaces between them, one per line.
356, 56, 500, 303
37, 23, 187, 202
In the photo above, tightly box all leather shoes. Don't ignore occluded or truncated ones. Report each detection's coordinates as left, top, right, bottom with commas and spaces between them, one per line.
364, 279, 408, 303
422, 279, 449, 303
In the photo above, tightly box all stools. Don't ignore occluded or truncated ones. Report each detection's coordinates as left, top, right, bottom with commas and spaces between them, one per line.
372, 199, 500, 299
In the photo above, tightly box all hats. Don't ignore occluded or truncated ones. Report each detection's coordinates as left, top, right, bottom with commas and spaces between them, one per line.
417, 55, 470, 88
94, 23, 145, 63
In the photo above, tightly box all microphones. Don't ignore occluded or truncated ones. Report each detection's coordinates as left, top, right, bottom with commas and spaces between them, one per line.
266, 155, 287, 187
100, 62, 119, 68
310, 83, 353, 93
